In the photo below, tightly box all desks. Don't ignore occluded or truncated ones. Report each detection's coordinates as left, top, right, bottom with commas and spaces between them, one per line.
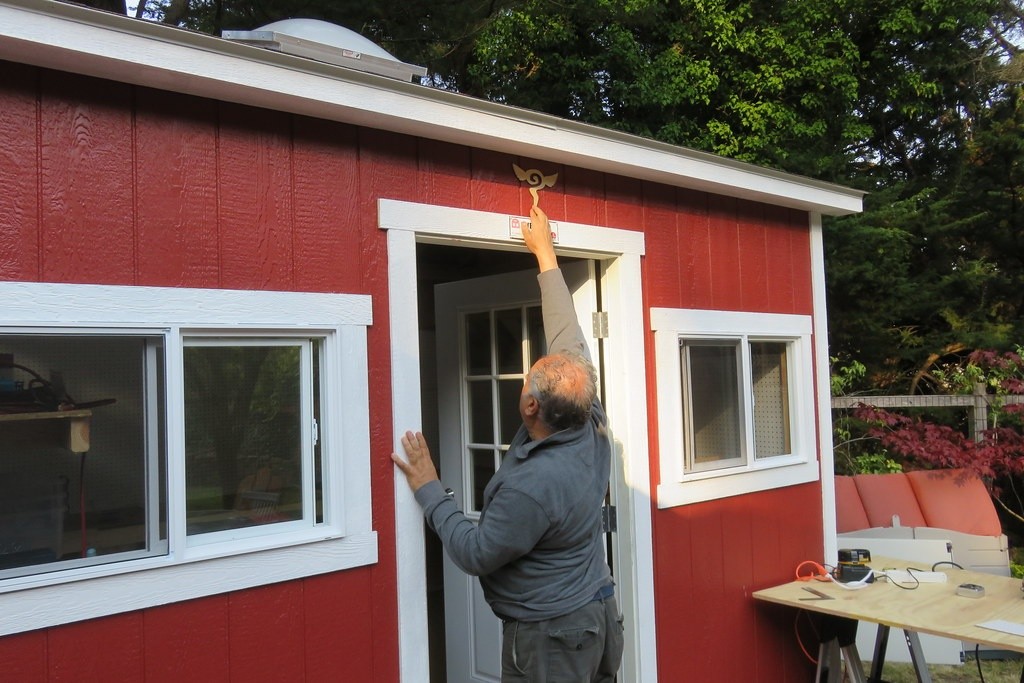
752, 557, 1024, 683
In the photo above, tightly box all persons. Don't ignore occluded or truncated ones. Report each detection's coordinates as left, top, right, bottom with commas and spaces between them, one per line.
389, 205, 625, 682
